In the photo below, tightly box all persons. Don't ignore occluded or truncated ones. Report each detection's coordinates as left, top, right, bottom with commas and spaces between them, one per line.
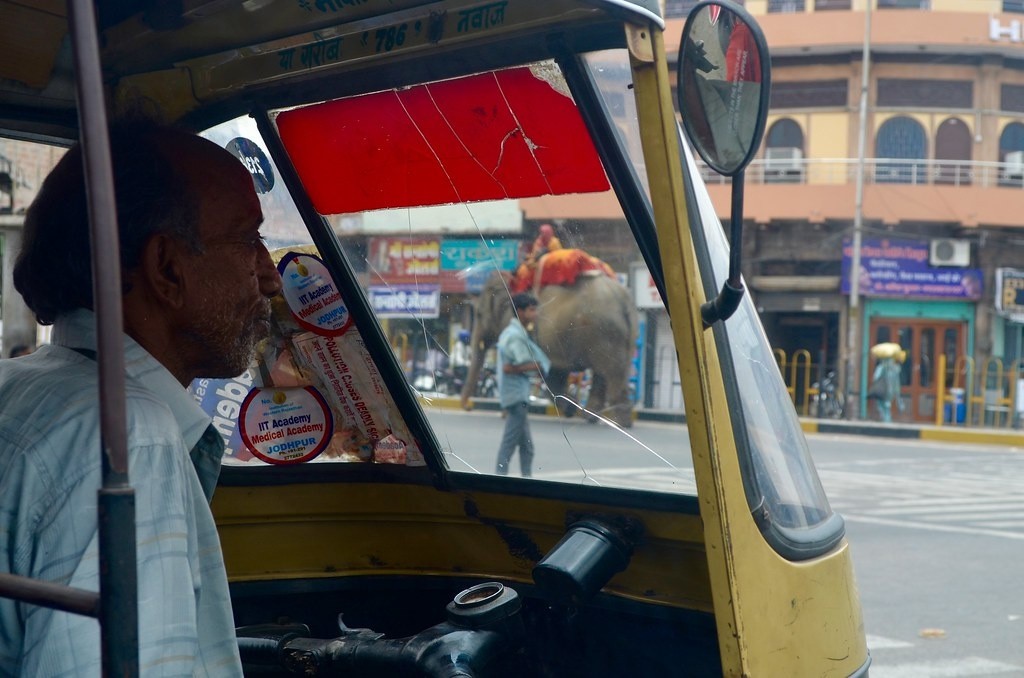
872, 356, 906, 423
449, 330, 473, 380
493, 293, 550, 476
522, 221, 562, 272
0, 116, 283, 676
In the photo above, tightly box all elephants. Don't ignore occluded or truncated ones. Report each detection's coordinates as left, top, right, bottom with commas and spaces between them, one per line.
459, 249, 640, 430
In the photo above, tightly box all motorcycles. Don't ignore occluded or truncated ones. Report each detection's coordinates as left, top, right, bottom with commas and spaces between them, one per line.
1, 1, 873, 678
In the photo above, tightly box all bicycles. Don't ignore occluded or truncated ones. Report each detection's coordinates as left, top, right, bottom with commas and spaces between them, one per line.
812, 371, 845, 418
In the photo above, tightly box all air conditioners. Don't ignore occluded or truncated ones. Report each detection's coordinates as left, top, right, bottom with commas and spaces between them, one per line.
765, 147, 802, 172
1005, 151, 1024, 178
928, 238, 970, 267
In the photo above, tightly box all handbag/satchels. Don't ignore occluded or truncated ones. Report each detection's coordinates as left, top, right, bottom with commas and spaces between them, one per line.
867, 362, 888, 400
